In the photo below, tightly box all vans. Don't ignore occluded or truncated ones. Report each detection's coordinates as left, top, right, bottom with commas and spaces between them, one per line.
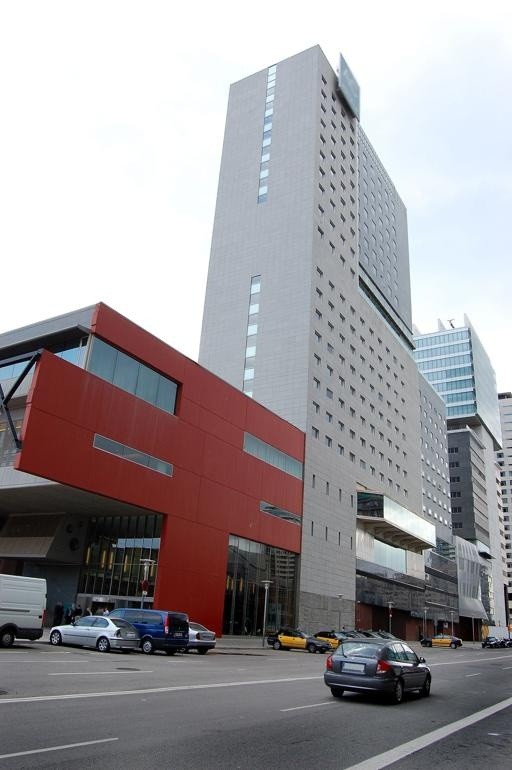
0, 574, 47, 647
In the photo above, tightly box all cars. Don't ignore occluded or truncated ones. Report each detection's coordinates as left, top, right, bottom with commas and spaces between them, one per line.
482, 637, 512, 648
421, 635, 463, 649
49, 608, 216, 654
267, 630, 431, 703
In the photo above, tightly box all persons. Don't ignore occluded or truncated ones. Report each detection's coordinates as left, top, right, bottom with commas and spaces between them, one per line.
51, 599, 110, 627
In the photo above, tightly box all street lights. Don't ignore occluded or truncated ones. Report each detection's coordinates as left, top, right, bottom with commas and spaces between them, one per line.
140, 559, 156, 608
386, 601, 475, 647
336, 593, 344, 632
261, 580, 274, 647
